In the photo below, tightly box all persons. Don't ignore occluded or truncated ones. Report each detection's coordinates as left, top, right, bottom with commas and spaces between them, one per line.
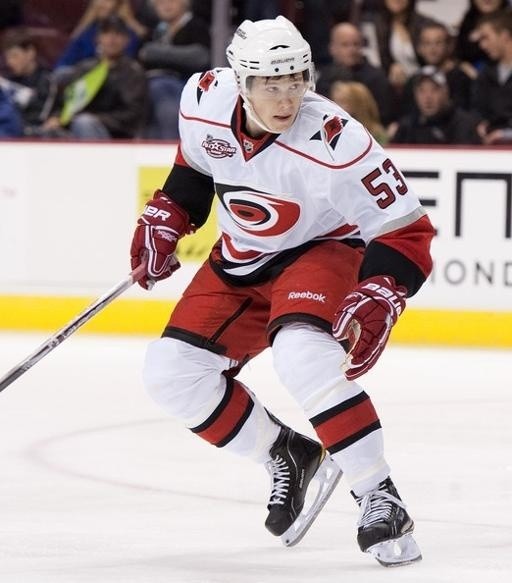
131, 13, 435, 550
1, 1, 512, 148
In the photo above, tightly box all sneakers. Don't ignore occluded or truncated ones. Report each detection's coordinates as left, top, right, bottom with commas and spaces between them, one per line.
351, 475, 414, 550
263, 407, 323, 537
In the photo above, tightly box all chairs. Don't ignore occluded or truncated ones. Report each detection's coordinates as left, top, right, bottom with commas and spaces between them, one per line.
0, 0, 512, 143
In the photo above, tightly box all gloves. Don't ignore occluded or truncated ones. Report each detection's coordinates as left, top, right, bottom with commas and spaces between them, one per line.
330, 274, 407, 381
131, 189, 195, 290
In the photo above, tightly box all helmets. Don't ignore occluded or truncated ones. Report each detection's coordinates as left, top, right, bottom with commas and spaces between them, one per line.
225, 16, 312, 134
